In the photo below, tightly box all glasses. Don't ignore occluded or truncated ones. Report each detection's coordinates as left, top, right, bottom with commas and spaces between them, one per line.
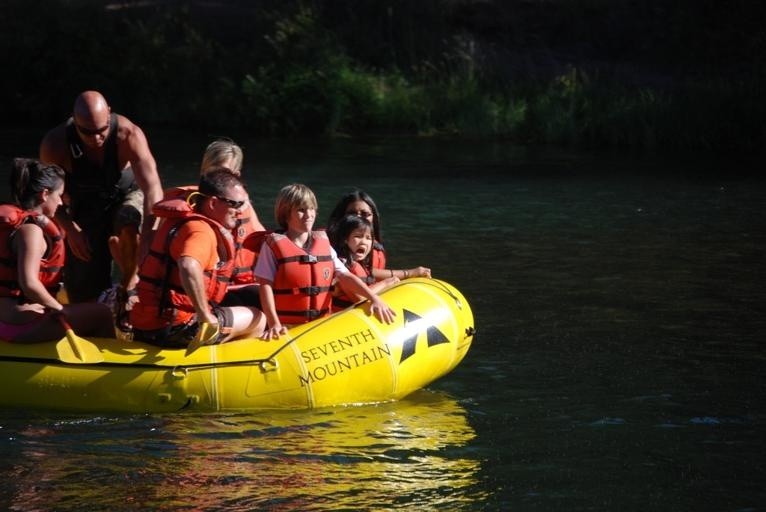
211, 193, 246, 209
72, 111, 111, 136
346, 209, 373, 219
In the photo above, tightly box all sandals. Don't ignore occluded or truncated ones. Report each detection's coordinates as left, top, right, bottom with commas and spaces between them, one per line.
116, 285, 140, 332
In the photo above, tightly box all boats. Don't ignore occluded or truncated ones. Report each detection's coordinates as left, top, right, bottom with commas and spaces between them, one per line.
0, 274, 476, 414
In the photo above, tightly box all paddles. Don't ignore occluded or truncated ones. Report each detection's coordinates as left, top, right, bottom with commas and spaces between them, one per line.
55, 311, 103, 363
185, 323, 220, 356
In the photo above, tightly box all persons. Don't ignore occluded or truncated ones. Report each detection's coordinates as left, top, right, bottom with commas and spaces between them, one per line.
39, 90, 165, 332
242, 181, 431, 341
155, 140, 270, 313
0, 157, 118, 343
129, 167, 266, 347
